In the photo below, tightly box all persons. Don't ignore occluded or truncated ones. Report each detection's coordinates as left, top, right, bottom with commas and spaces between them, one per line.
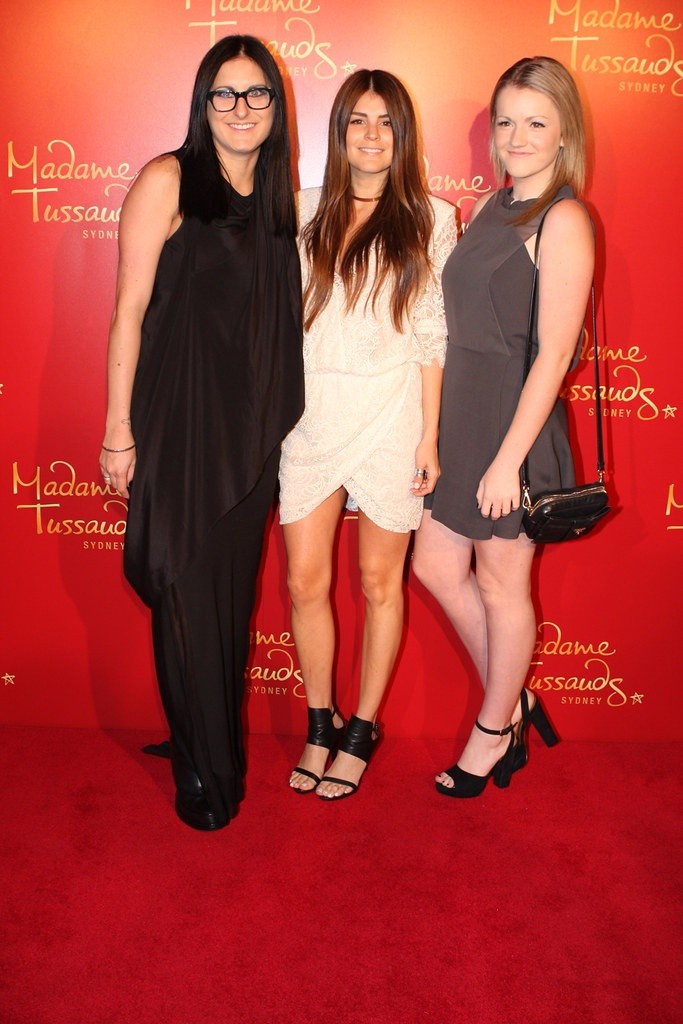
273, 68, 458, 809
91, 33, 308, 834
409, 54, 605, 801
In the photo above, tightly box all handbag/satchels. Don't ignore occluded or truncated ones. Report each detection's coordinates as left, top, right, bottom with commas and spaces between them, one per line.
525, 484, 610, 544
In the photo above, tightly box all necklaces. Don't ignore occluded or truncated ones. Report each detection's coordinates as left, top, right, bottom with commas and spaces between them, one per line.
350, 194, 383, 203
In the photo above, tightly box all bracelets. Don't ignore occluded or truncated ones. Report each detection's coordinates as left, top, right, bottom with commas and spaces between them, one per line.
101, 442, 136, 454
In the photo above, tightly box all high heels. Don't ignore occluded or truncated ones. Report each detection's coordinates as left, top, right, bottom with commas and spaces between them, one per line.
293, 706, 346, 793
489, 686, 559, 776
173, 750, 225, 830
316, 713, 379, 801
435, 720, 518, 798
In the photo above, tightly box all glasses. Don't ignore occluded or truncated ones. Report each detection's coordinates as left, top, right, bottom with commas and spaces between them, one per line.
206, 87, 276, 112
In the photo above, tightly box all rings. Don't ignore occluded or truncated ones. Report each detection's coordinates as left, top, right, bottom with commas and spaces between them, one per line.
103, 476, 111, 480
413, 467, 424, 477
